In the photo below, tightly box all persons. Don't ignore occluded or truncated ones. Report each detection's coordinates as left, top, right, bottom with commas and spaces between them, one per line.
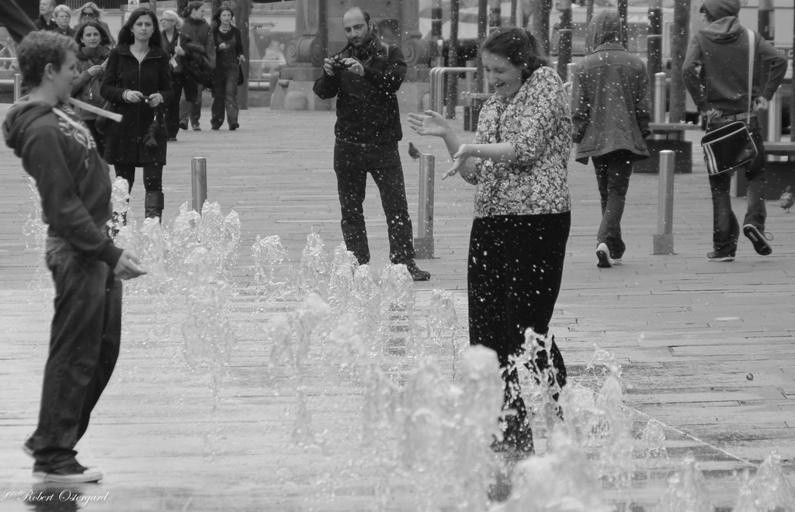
160, 10, 194, 141
681, 0, 788, 262
99, 6, 175, 235
208, 6, 246, 130
2, 31, 146, 485
408, 27, 574, 453
313, 8, 431, 280
33, 0, 116, 159
180, 3, 216, 130
569, 11, 651, 267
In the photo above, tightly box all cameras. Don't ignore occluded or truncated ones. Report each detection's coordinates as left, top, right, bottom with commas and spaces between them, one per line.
331, 54, 346, 70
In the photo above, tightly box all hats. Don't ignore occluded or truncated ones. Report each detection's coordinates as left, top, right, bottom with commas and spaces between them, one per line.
705, 0, 741, 19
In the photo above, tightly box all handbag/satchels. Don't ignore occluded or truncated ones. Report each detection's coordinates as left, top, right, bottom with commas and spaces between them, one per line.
94, 100, 116, 134
701, 120, 761, 174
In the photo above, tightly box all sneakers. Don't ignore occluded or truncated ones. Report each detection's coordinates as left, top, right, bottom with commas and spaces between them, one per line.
392, 259, 430, 282
22, 435, 102, 483
707, 251, 734, 262
180, 116, 239, 131
597, 243, 615, 268
743, 223, 773, 255
